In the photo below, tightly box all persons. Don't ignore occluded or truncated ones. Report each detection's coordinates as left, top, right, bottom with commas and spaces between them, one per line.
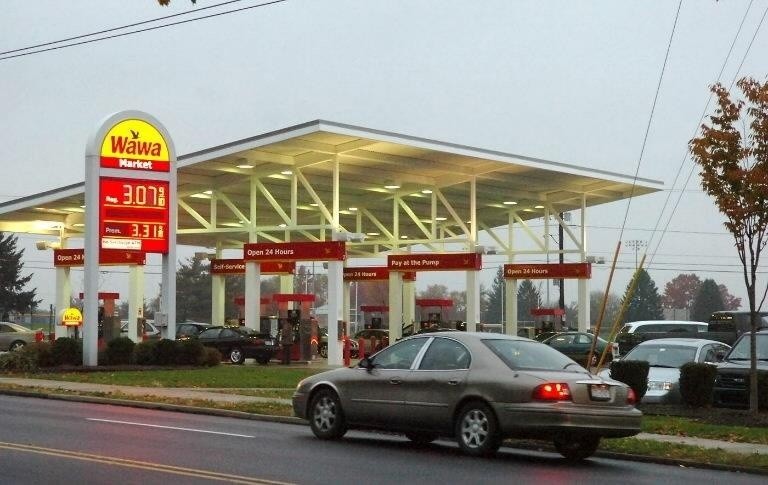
280, 318, 293, 366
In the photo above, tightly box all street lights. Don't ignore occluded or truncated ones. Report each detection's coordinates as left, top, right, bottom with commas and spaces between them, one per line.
624, 240, 647, 270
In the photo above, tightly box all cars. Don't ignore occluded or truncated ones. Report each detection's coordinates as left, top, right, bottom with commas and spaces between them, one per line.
533, 310, 768, 408
79, 319, 280, 366
292, 330, 642, 459
319, 324, 458, 358
0, 320, 43, 352
